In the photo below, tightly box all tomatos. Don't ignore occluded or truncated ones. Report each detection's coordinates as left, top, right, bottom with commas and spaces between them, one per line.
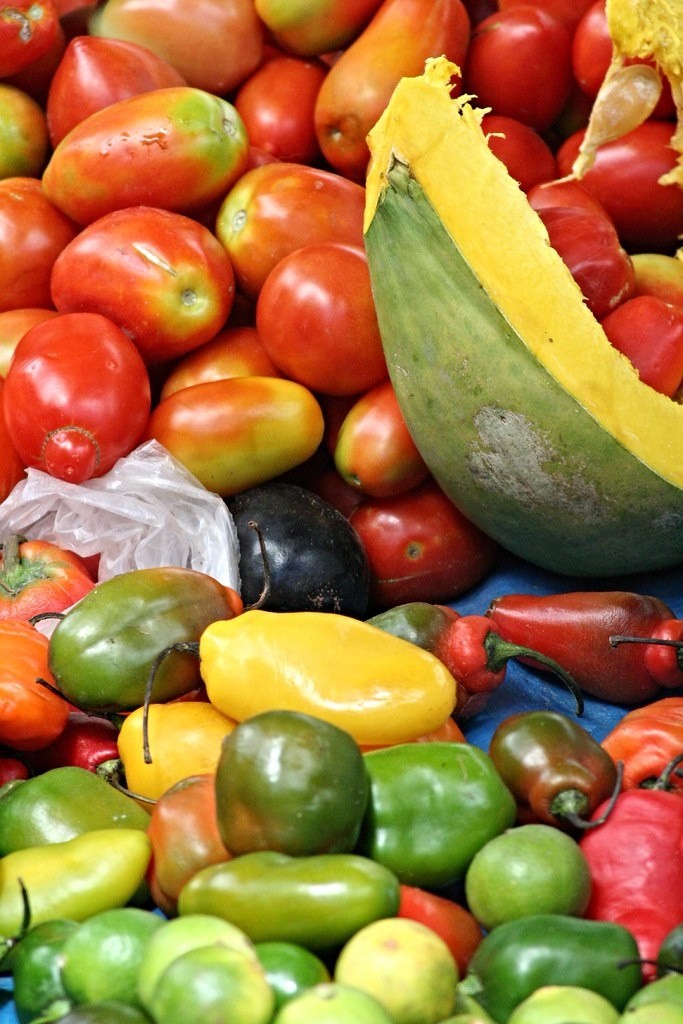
0, 0, 683, 596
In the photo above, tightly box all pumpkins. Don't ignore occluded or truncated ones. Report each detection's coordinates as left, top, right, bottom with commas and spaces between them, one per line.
364, 59, 682, 579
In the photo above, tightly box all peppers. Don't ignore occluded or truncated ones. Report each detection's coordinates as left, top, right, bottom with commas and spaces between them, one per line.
0, 522, 683, 1024
